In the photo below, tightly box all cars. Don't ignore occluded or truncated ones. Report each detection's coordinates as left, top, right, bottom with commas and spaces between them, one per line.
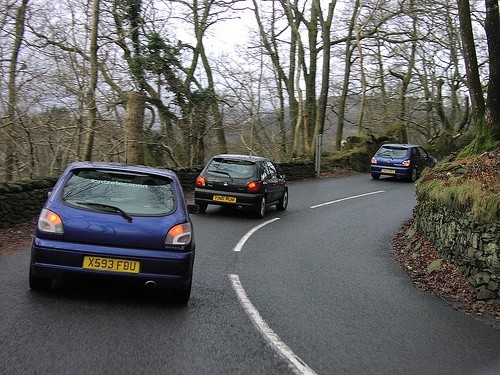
29, 161, 200, 306
369, 144, 436, 182
194, 154, 289, 218
